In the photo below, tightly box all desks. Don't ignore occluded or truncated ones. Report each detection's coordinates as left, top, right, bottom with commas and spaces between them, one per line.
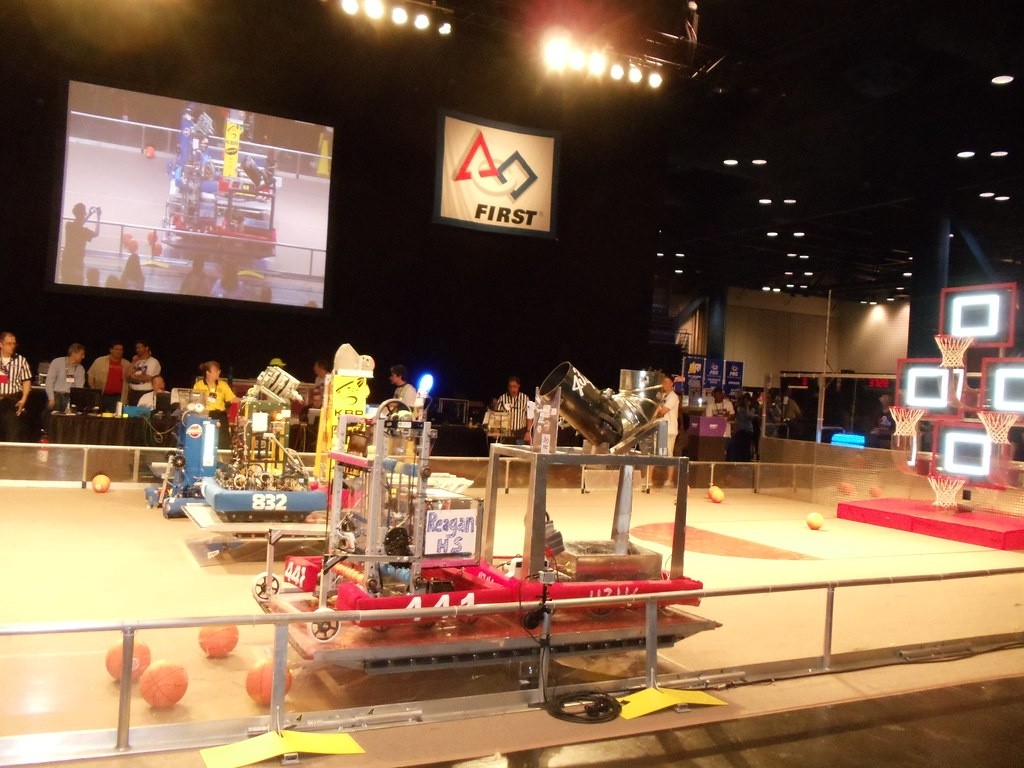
50, 409, 232, 449
688, 434, 726, 462
680, 406, 706, 413
430, 428, 489, 457
288, 424, 319, 453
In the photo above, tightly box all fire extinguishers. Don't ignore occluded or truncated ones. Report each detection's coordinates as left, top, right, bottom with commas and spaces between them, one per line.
36, 428, 49, 462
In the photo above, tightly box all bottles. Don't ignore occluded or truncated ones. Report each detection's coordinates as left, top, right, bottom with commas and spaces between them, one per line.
469, 418, 473, 428
116, 402, 122, 417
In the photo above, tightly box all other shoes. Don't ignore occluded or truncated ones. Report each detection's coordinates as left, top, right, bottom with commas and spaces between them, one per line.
663, 480, 674, 487
642, 478, 653, 487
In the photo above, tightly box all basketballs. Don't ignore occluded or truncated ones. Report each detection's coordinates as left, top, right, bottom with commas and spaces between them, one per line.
806, 512, 824, 530
707, 485, 724, 503
841, 483, 854, 495
869, 486, 881, 497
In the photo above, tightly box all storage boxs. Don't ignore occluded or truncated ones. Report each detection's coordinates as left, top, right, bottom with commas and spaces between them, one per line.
688, 415, 727, 437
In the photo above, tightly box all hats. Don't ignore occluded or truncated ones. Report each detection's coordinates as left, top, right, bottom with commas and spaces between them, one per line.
270, 358, 287, 367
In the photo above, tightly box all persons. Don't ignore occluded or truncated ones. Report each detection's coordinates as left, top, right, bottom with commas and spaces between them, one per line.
483, 376, 533, 445
62, 204, 273, 301
41, 343, 85, 433
0, 332, 33, 441
873, 393, 894, 440
194, 360, 242, 448
390, 365, 417, 412
658, 376, 679, 456
705, 387, 803, 463
300, 361, 329, 451
87, 340, 161, 410
139, 375, 170, 410
270, 359, 286, 393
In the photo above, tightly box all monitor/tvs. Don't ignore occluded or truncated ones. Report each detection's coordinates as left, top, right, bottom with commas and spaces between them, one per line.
70, 387, 102, 416
